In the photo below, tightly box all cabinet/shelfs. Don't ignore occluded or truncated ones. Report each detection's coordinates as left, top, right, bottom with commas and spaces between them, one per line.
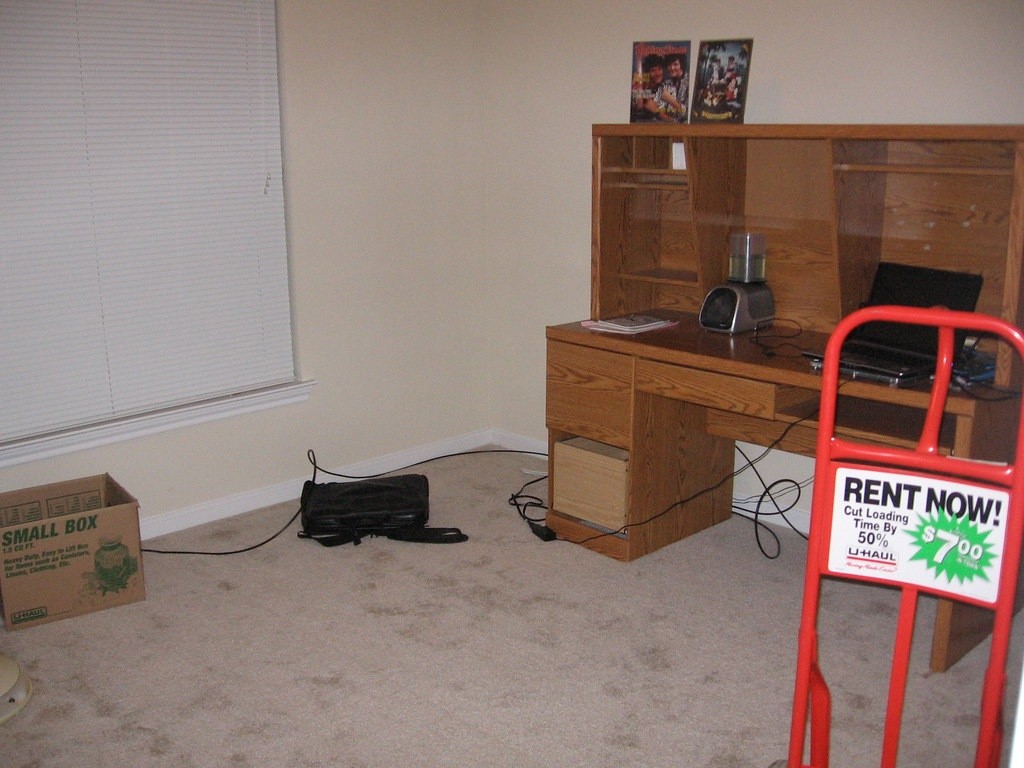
546, 122, 1024, 674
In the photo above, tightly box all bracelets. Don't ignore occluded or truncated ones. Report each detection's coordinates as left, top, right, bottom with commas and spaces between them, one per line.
655, 107, 663, 117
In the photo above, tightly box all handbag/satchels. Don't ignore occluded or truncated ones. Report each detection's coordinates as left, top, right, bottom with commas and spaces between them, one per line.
297, 474, 468, 548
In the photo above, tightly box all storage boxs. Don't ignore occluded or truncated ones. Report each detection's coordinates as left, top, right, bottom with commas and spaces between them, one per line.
0, 473, 148, 631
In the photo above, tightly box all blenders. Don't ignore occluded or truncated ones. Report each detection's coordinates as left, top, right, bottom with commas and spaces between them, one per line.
696, 231, 777, 334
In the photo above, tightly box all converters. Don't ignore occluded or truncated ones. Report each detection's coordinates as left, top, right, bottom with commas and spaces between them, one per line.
532, 525, 556, 541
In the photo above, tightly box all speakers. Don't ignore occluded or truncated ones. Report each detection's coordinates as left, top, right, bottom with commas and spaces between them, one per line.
697, 280, 776, 336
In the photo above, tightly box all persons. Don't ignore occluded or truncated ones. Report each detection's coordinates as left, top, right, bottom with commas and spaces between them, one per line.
632, 53, 689, 125
699, 55, 743, 112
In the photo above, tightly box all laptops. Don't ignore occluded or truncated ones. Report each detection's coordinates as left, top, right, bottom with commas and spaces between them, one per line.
801, 261, 984, 378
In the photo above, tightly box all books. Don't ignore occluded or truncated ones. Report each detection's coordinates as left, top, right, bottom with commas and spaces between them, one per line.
588, 314, 680, 335
